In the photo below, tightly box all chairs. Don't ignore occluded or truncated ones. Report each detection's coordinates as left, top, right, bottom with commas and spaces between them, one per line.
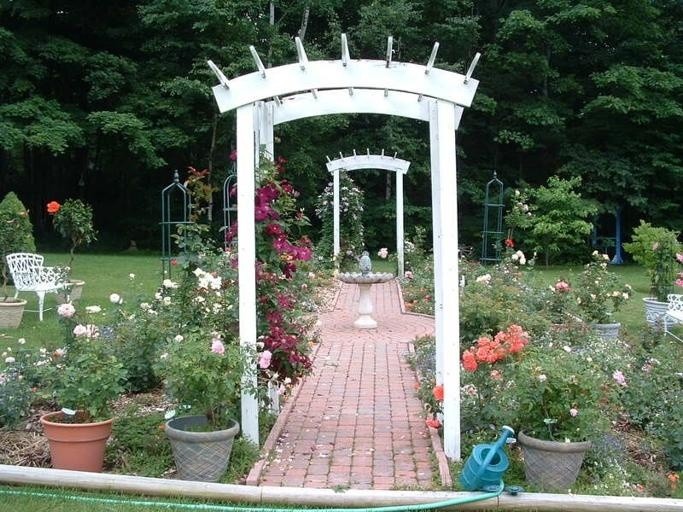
4, 253, 68, 322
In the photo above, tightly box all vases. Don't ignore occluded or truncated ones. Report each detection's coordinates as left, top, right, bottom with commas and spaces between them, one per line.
587, 322, 621, 346
644, 297, 679, 335
160, 414, 240, 482
54, 279, 86, 305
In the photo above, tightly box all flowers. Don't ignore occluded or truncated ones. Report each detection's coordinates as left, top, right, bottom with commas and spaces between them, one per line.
152, 325, 281, 422
568, 251, 634, 323
46, 200, 96, 282
501, 347, 606, 441
621, 220, 682, 302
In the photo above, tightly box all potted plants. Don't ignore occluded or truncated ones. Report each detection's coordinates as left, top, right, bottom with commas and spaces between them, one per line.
31, 337, 126, 473
517, 431, 591, 493
0, 211, 29, 330
